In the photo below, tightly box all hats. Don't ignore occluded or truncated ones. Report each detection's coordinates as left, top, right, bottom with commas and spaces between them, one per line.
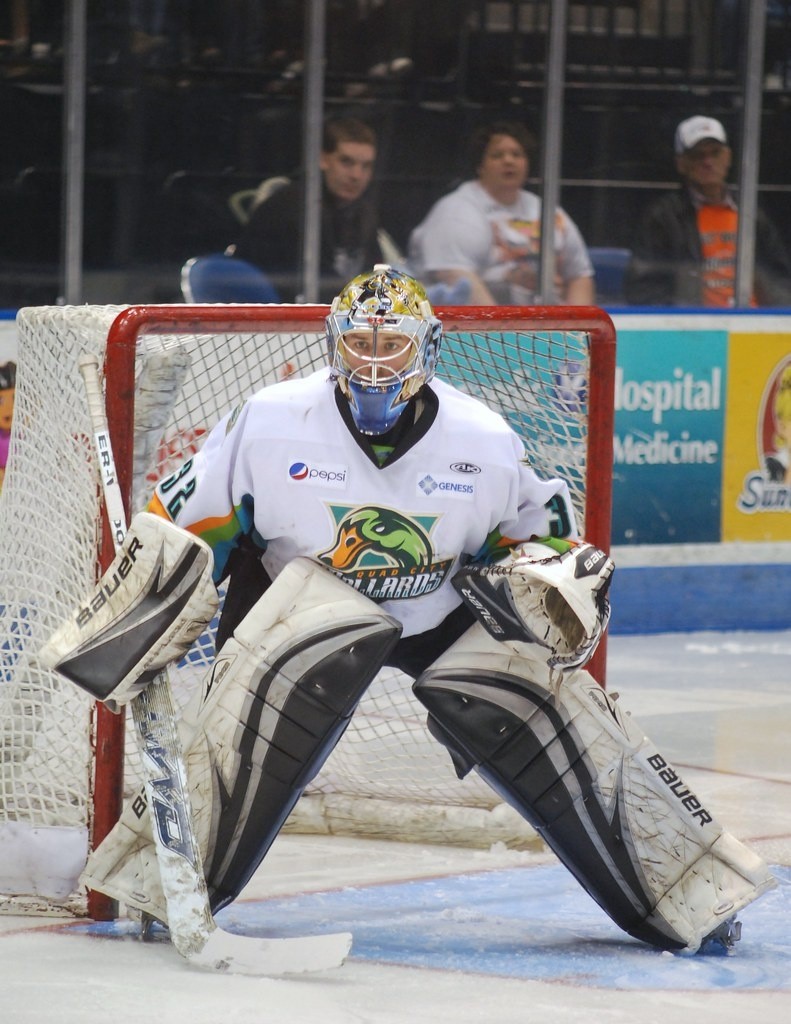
671, 116, 727, 167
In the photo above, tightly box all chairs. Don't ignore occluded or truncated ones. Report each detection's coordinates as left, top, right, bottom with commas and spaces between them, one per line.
180, 253, 279, 304
586, 246, 631, 307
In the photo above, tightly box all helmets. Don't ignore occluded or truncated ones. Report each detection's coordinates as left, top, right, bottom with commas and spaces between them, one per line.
331, 269, 441, 436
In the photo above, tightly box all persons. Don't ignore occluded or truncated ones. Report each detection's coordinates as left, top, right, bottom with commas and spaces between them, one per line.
408, 121, 594, 306
190, 118, 379, 303
40, 268, 777, 958
624, 116, 791, 307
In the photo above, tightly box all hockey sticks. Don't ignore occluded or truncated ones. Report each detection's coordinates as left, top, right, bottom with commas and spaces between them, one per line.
75, 350, 354, 979
